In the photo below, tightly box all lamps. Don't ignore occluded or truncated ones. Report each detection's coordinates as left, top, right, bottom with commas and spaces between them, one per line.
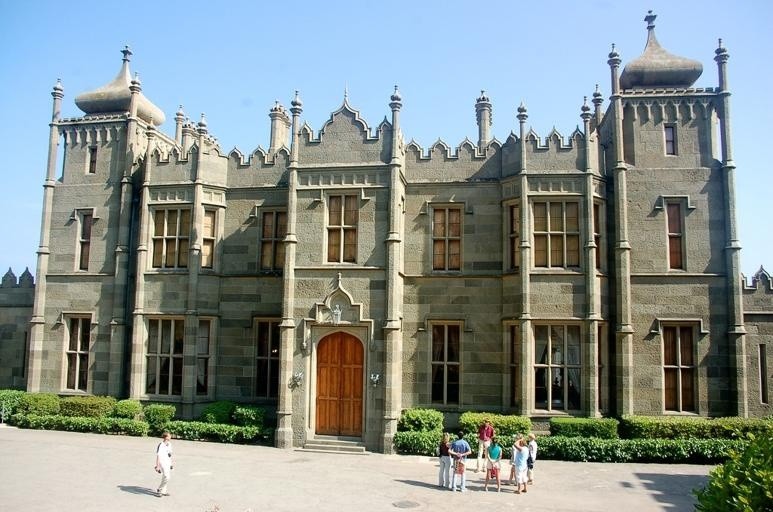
290, 371, 304, 387
332, 303, 342, 326
370, 372, 380, 387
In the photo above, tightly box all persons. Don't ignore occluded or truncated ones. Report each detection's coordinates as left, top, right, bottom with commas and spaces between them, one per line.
439, 418, 536, 494
154, 431, 174, 495
